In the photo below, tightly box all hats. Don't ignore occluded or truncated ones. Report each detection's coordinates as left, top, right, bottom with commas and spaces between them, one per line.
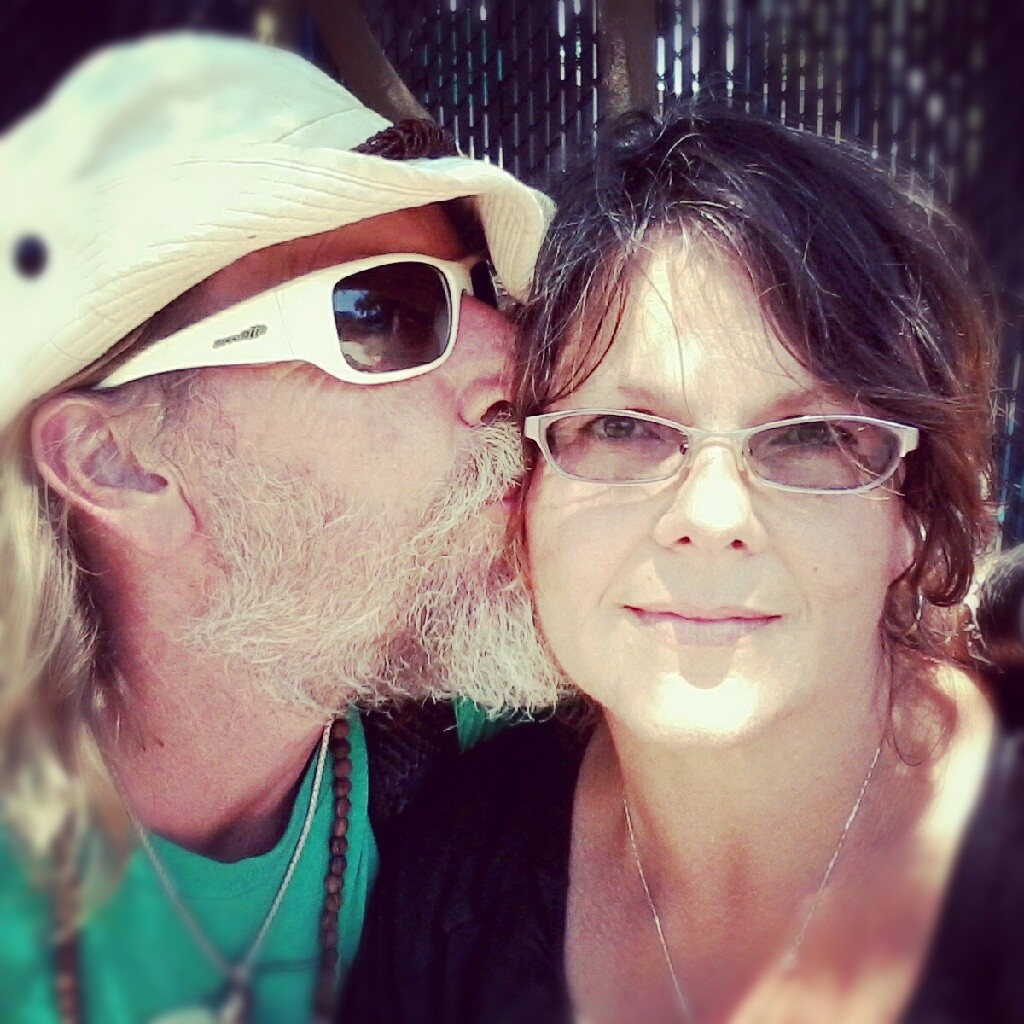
0, 32, 559, 442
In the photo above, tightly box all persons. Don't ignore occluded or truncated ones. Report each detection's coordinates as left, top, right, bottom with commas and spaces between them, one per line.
0, 33, 566, 1024
341, 112, 1024, 1024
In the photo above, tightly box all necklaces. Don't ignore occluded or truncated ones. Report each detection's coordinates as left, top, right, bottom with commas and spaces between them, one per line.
57, 710, 351, 1024
624, 645, 894, 1024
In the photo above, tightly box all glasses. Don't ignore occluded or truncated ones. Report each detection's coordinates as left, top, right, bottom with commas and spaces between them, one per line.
522, 408, 918, 496
90, 253, 515, 392
